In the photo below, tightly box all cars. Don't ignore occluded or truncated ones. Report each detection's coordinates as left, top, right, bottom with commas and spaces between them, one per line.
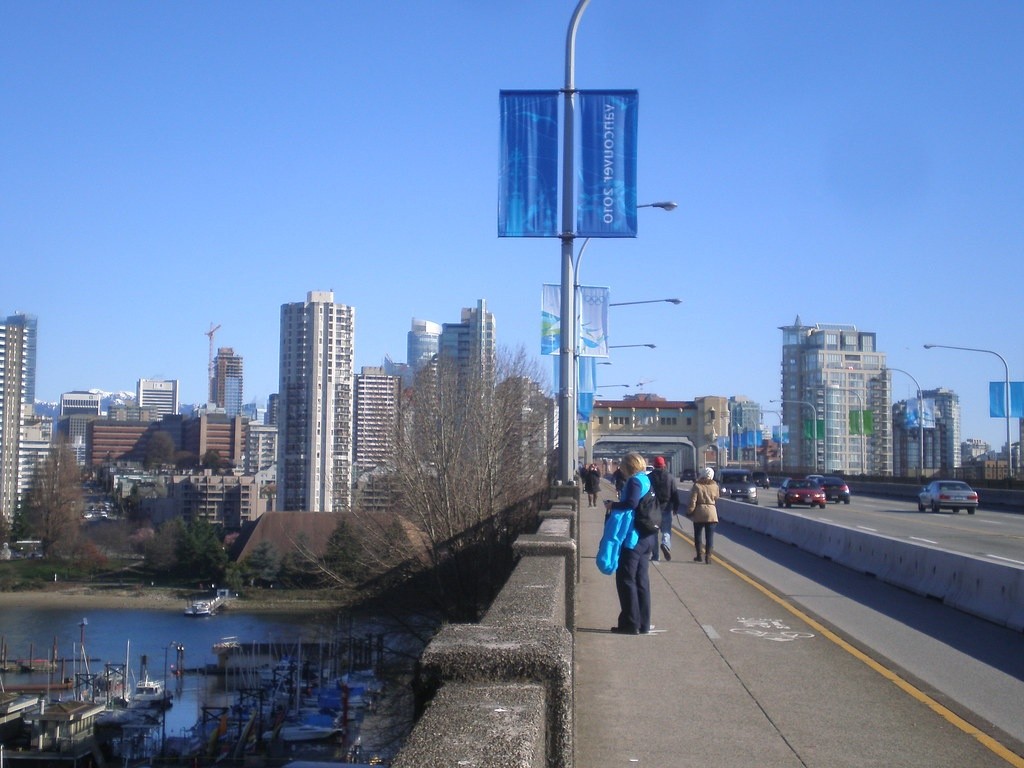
680, 469, 695, 482
816, 477, 850, 504
777, 478, 826, 510
919, 480, 978, 514
645, 466, 654, 475
806, 474, 824, 482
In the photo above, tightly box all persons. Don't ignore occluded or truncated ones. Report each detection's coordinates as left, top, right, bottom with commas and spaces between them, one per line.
603, 452, 657, 634
579, 463, 601, 508
647, 456, 679, 561
739, 474, 749, 482
610, 465, 626, 499
805, 482, 812, 488
686, 467, 719, 564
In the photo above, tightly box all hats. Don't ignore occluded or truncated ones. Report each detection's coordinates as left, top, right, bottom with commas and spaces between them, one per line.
653, 456, 665, 470
702, 467, 715, 481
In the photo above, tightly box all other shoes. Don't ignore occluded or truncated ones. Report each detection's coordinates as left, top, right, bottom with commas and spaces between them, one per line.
661, 545, 672, 562
611, 622, 648, 635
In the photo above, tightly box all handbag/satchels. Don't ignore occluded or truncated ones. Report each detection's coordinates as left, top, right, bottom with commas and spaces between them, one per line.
635, 488, 662, 535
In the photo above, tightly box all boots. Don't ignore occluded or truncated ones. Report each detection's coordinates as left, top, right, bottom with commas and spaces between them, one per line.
694, 543, 705, 562
705, 545, 713, 565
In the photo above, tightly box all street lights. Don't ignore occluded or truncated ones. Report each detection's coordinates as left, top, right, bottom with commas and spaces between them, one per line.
741, 407, 783, 472
571, 200, 678, 474
770, 399, 818, 473
924, 344, 1013, 477
806, 387, 863, 475
879, 368, 923, 477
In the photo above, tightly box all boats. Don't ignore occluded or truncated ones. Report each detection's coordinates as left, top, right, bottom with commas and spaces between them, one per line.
0, 659, 172, 768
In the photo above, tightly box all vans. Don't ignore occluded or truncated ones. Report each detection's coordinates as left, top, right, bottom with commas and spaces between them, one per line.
751, 471, 770, 489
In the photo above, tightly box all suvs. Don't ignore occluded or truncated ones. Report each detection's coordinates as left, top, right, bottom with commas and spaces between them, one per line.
714, 468, 758, 504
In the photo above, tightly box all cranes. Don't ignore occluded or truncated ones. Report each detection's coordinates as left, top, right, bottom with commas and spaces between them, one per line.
204, 322, 221, 402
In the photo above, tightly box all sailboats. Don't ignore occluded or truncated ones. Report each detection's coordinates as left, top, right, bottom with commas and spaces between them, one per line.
171, 616, 377, 768
